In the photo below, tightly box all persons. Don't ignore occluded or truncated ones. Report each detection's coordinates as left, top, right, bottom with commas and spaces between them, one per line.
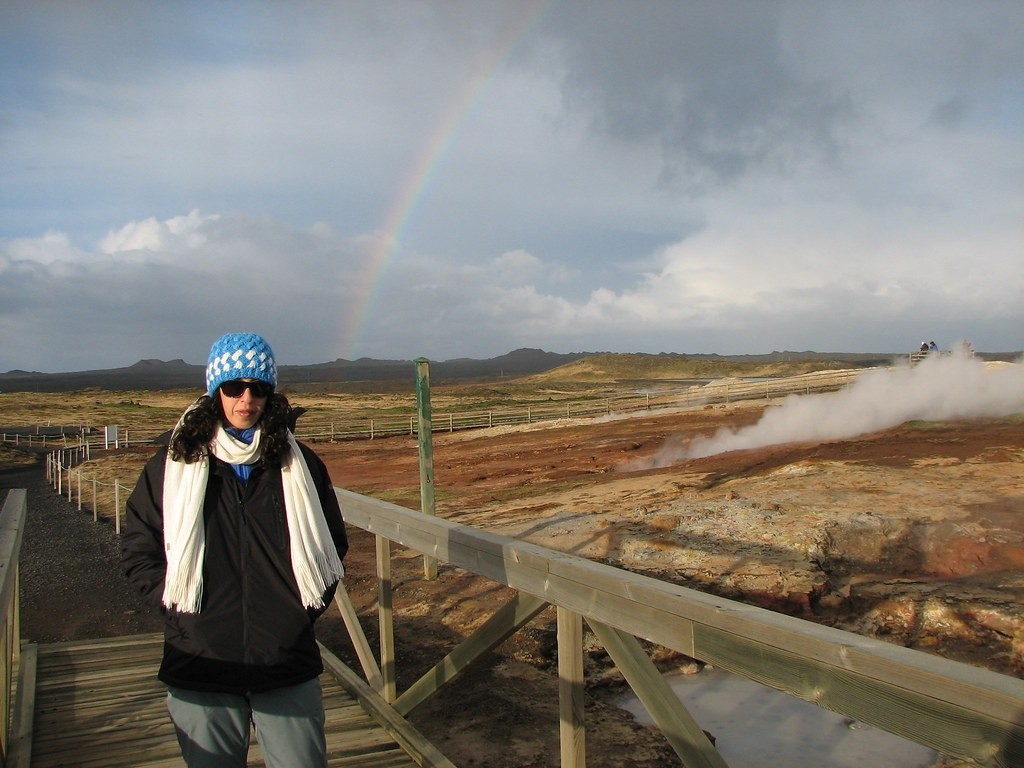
118, 331, 348, 768
929, 341, 938, 351
919, 340, 928, 355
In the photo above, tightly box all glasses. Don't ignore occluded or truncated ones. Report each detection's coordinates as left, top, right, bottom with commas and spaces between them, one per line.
219, 380, 274, 398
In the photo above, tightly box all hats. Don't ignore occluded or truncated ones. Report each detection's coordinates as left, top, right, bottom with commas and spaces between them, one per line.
206, 333, 278, 398
